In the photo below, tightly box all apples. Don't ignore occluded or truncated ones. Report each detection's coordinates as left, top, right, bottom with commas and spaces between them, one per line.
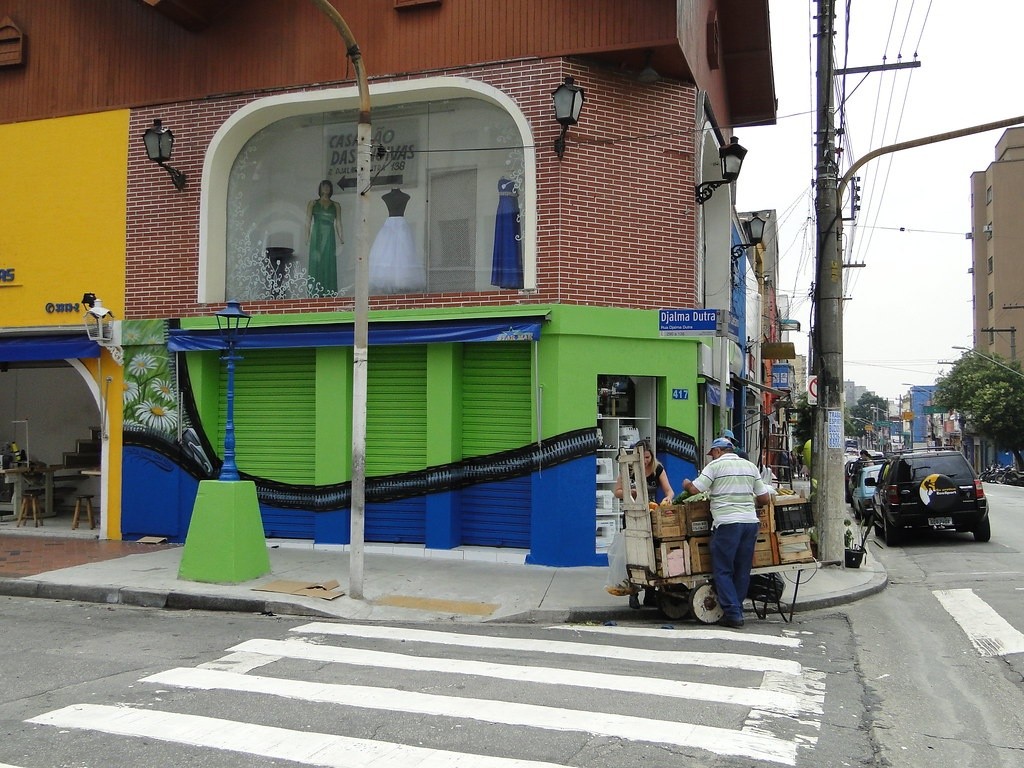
650, 500, 674, 513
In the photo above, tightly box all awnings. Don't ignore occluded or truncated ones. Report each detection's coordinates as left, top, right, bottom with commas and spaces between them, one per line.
897, 432, 910, 435
761, 342, 795, 360
732, 372, 793, 429
875, 421, 893, 427
785, 408, 799, 413
779, 319, 800, 331
787, 419, 800, 423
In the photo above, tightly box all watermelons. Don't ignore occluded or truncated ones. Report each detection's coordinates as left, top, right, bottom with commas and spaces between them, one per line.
672, 490, 690, 505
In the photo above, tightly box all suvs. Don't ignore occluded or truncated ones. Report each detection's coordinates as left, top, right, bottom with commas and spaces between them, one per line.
864, 445, 993, 547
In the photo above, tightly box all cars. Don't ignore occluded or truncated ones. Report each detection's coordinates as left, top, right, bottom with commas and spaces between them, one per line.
844, 449, 891, 527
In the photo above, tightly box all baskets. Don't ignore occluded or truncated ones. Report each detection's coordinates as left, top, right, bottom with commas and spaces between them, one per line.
774, 502, 814, 532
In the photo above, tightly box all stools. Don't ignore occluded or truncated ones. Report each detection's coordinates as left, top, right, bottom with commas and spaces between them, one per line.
72, 494, 96, 530
16, 492, 44, 528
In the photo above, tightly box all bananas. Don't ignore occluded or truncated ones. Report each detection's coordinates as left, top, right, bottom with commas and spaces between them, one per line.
777, 489, 796, 495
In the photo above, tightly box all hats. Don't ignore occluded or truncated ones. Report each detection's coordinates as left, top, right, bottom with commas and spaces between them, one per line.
706, 438, 733, 455
725, 430, 739, 443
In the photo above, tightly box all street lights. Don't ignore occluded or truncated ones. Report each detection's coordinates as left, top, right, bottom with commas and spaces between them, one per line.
870, 405, 891, 452
854, 417, 879, 452
901, 381, 975, 470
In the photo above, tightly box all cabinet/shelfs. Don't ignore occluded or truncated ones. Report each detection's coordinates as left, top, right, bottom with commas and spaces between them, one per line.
596, 417, 650, 547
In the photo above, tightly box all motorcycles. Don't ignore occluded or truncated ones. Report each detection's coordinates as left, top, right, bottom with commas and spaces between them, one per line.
978, 460, 1024, 485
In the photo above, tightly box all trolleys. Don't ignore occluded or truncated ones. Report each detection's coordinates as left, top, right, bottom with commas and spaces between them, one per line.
612, 442, 844, 626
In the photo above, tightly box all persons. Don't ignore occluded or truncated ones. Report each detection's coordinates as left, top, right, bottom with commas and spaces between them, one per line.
781, 449, 804, 482
968, 455, 971, 463
369, 188, 425, 293
490, 170, 524, 289
615, 440, 674, 609
990, 460, 1004, 468
305, 180, 344, 298
860, 449, 874, 467
724, 430, 750, 462
682, 438, 769, 629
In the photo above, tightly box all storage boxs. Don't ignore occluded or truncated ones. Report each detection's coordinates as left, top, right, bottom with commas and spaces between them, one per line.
651, 494, 815, 576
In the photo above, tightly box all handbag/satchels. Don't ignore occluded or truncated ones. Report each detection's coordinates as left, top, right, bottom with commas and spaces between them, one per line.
746, 572, 786, 603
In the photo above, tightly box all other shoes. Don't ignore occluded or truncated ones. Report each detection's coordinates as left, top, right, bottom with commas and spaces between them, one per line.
719, 620, 744, 628
629, 595, 640, 609
643, 597, 658, 608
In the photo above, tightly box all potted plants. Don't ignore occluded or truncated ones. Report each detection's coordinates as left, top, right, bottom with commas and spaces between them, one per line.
844, 516, 884, 567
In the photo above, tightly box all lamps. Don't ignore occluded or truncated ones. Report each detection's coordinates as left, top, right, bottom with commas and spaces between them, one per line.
731, 212, 767, 267
694, 137, 747, 206
82, 299, 115, 341
550, 77, 586, 157
142, 119, 187, 191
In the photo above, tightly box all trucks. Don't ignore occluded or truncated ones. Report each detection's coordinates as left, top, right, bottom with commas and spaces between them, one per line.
844, 439, 859, 456
